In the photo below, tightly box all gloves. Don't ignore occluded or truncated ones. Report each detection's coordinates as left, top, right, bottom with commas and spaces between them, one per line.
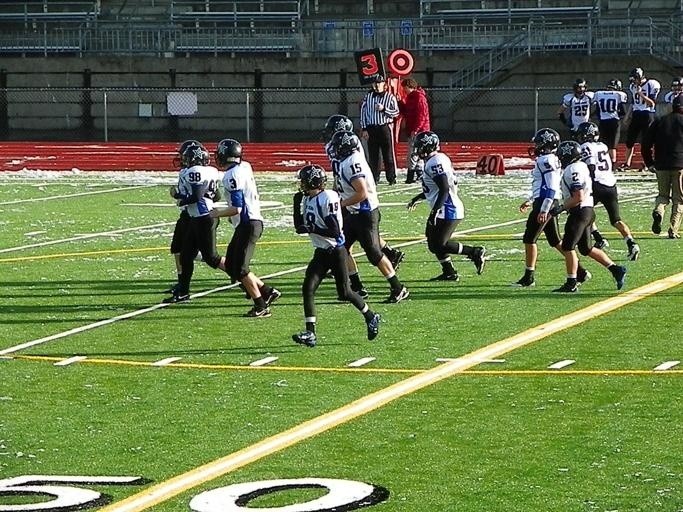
407, 198, 418, 210
296, 221, 318, 234
429, 209, 440, 226
294, 192, 303, 206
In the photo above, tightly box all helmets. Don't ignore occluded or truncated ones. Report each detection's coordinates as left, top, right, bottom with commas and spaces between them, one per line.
531, 122, 600, 168
629, 68, 644, 85
325, 115, 359, 160
671, 77, 683, 108
573, 79, 587, 92
607, 79, 622, 90
415, 131, 440, 159
179, 140, 242, 166
297, 165, 327, 195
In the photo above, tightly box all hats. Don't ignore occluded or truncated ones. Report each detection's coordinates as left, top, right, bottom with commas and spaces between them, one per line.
371, 75, 384, 83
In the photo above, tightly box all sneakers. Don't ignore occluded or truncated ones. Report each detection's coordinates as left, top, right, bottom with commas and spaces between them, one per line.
552, 270, 591, 292
627, 243, 640, 260
615, 266, 626, 290
473, 247, 485, 274
337, 249, 409, 303
368, 313, 380, 340
430, 271, 459, 281
243, 288, 282, 317
594, 239, 609, 249
617, 163, 632, 170
292, 331, 316, 347
512, 277, 535, 286
163, 288, 190, 303
652, 212, 680, 239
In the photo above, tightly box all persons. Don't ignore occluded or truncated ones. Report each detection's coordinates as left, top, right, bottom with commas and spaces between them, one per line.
396, 79, 430, 185
292, 165, 380, 348
162, 140, 202, 294
512, 68, 683, 295
208, 138, 282, 318
406, 130, 486, 282
330, 130, 410, 304
321, 115, 404, 280
162, 144, 251, 305
361, 75, 401, 187
